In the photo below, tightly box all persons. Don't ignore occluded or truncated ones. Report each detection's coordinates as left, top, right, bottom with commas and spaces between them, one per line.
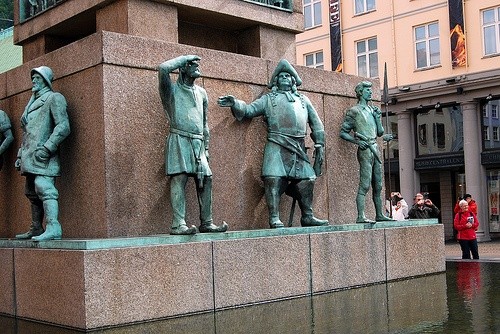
458, 262, 481, 302
158, 55, 228, 233
454, 197, 463, 242
218, 59, 328, 227
385, 191, 408, 220
14, 65, 71, 242
453, 198, 479, 259
463, 194, 478, 231
0, 111, 14, 155
341, 80, 398, 223
383, 134, 393, 141
405, 192, 440, 218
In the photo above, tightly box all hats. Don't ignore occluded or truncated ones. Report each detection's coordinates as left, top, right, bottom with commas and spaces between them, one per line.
464, 194, 472, 200
392, 195, 403, 205
